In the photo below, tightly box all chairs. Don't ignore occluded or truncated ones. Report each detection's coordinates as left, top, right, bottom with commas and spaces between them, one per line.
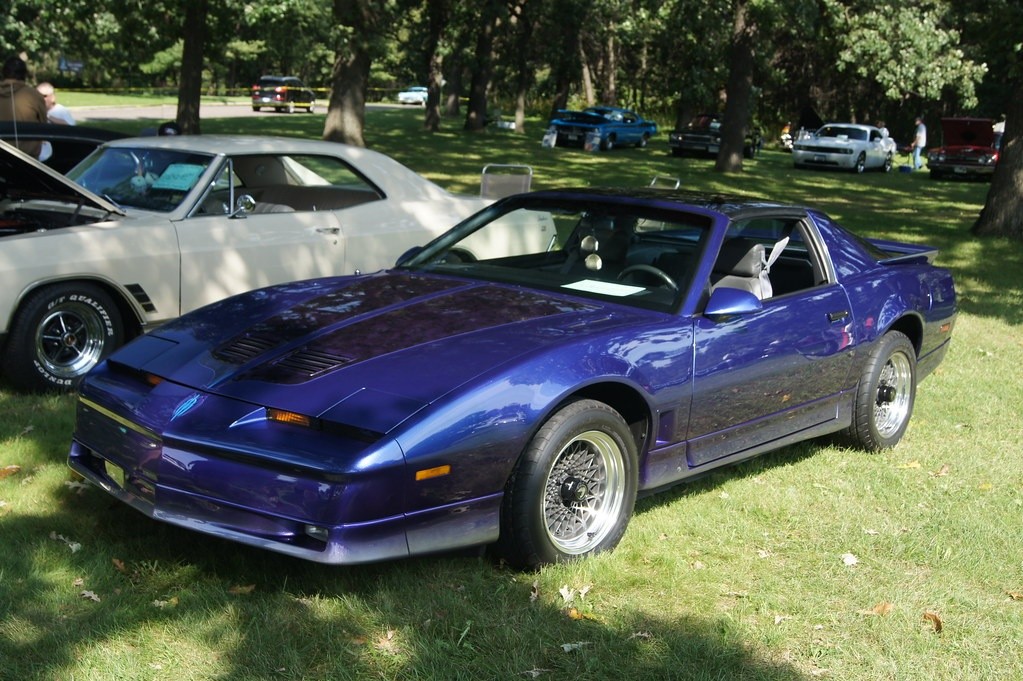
562, 226, 635, 286
636, 174, 680, 232
480, 163, 533, 200
238, 201, 293, 214
706, 236, 773, 301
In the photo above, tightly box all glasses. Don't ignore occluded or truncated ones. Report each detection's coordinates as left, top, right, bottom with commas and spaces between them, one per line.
44, 94, 46, 98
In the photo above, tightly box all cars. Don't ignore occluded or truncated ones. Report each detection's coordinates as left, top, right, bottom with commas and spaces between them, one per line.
789, 122, 897, 173
926, 117, 1000, 180
667, 111, 766, 158
542, 103, 658, 153
250, 75, 317, 112
394, 86, 429, 108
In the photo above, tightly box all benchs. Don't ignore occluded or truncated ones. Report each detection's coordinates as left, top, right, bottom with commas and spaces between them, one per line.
252, 185, 377, 211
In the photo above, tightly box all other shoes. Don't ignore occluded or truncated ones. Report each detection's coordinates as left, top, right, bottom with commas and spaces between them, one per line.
914, 165, 922, 170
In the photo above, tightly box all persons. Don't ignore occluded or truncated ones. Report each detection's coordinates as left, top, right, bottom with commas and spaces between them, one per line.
0, 57, 47, 161
150, 121, 186, 177
781, 122, 796, 145
911, 117, 927, 169
36, 83, 75, 161
752, 127, 762, 156
876, 120, 889, 137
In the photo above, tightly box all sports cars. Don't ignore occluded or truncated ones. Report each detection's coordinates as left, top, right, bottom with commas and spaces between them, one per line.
0, 118, 557, 395
66, 178, 959, 569
